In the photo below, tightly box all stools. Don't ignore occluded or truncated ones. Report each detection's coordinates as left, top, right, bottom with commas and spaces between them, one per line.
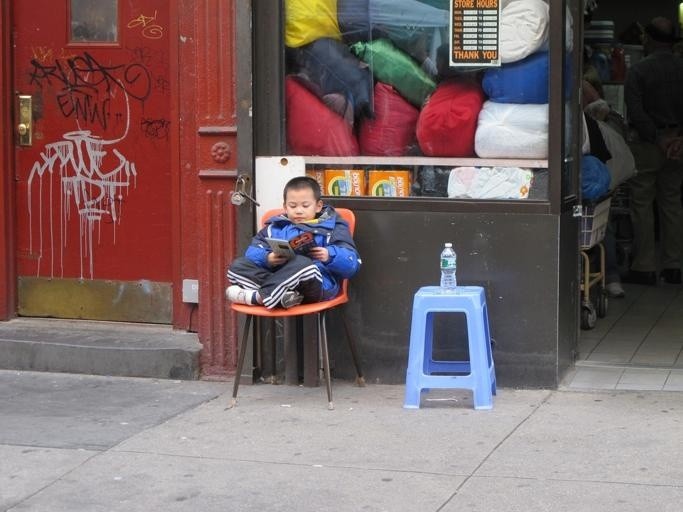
402, 285, 496, 411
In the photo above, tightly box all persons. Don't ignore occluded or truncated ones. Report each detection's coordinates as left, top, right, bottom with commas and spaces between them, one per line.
594, 221, 625, 298
224, 176, 362, 310
583, 44, 605, 101
621, 17, 683, 284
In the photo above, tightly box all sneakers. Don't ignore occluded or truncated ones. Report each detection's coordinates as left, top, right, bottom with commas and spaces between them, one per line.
605, 282, 625, 297
226, 285, 257, 306
280, 290, 304, 308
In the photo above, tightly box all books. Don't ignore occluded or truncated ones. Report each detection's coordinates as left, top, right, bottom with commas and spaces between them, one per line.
263, 231, 319, 260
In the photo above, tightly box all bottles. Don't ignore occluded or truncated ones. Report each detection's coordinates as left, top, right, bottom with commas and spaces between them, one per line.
439, 242, 457, 291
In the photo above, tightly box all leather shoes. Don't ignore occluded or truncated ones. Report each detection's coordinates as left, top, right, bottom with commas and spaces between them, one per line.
660, 268, 681, 284
621, 269, 656, 285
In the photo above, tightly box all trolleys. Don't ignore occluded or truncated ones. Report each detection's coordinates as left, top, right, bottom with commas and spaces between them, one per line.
577, 192, 614, 330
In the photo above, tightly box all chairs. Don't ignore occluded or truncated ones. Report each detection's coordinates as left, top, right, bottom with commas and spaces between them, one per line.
231, 208, 366, 410
